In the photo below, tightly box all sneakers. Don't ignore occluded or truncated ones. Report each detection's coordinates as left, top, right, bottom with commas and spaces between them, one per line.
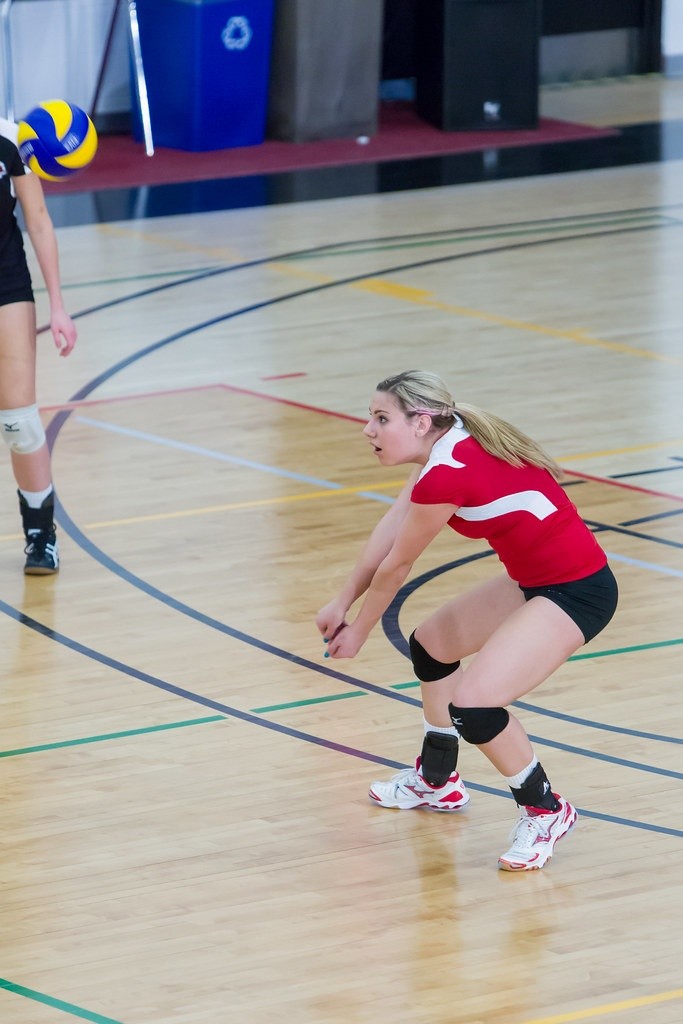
24, 523, 59, 575
497, 793, 579, 871
369, 755, 471, 812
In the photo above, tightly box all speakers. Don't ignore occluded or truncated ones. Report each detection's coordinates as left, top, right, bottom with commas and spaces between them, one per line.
412, 0, 538, 133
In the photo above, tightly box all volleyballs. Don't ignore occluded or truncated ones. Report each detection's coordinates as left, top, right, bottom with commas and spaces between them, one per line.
19, 98, 101, 181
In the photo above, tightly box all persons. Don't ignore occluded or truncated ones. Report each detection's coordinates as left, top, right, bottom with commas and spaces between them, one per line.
316, 369, 618, 873
1, 117, 76, 575
